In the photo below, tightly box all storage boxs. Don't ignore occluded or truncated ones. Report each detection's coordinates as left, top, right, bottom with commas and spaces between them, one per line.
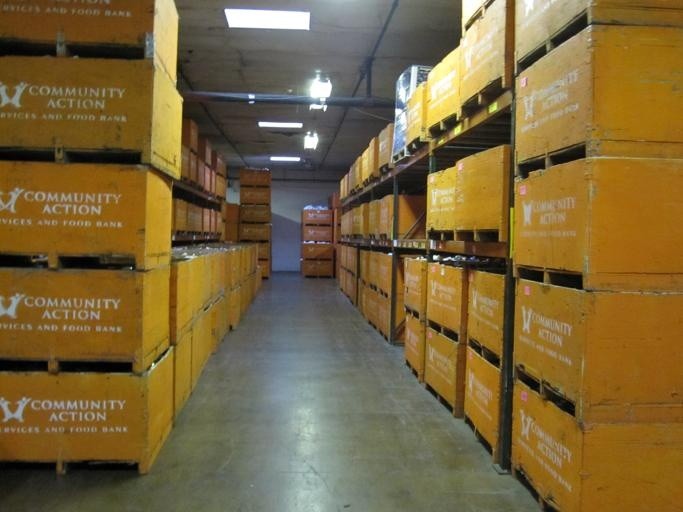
426, 49, 461, 132
0, 270, 172, 369
0, 56, 182, 185
0, 158, 173, 270
460, 0, 492, 31
173, 120, 238, 243
2, 0, 178, 82
303, 241, 331, 260
461, 3, 515, 113
515, 1, 680, 68
467, 267, 508, 362
239, 168, 272, 186
514, 277, 681, 422
304, 225, 332, 243
304, 207, 332, 226
428, 257, 467, 340
303, 260, 332, 279
175, 241, 270, 415
0, 369, 172, 476
426, 165, 457, 241
240, 185, 271, 204
454, 145, 510, 245
514, 25, 678, 175
462, 345, 500, 463
425, 322, 466, 418
514, 158, 681, 290
241, 204, 272, 222
332, 72, 426, 387
239, 220, 270, 241
512, 379, 680, 509
